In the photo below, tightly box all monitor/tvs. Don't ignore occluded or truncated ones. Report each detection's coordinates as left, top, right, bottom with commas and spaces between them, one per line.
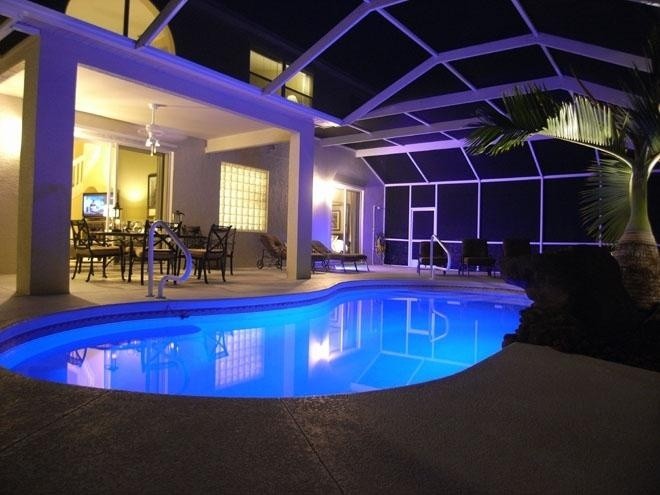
83, 193, 120, 219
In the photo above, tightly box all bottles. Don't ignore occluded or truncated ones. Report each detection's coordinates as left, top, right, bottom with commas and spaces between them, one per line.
90, 200, 96, 212
123, 220, 142, 232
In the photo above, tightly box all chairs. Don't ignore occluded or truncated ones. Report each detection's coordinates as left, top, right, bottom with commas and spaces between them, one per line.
71, 217, 237, 285
417, 235, 533, 278
258, 231, 369, 277
